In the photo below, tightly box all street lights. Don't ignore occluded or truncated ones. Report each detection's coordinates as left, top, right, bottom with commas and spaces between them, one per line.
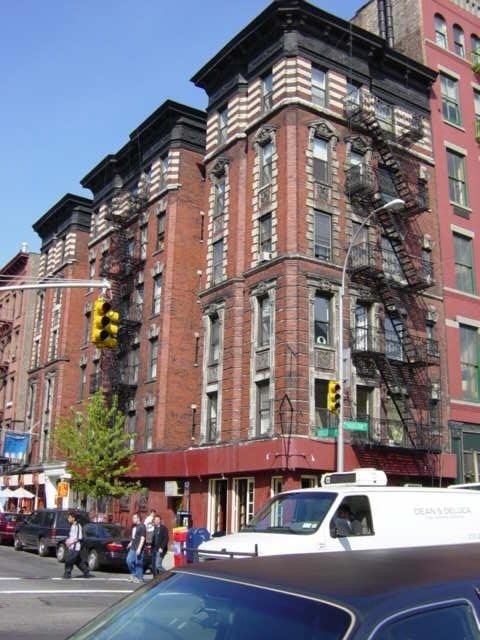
336, 198, 406, 472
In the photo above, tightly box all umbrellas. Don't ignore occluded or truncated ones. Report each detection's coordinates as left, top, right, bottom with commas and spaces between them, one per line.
9, 487, 36, 507
1, 486, 15, 504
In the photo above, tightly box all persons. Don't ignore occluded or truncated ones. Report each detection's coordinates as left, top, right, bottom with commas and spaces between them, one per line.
126, 512, 146, 583
144, 510, 157, 533
151, 516, 170, 580
337, 505, 364, 535
61, 513, 90, 578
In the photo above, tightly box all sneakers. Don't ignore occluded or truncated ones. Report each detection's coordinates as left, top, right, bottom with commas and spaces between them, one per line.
128, 576, 144, 584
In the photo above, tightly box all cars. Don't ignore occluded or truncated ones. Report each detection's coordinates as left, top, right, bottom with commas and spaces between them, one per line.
66, 542, 480, 640
0, 508, 152, 572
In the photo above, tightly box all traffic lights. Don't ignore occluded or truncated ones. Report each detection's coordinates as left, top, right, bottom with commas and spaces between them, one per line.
327, 380, 341, 412
90, 298, 120, 349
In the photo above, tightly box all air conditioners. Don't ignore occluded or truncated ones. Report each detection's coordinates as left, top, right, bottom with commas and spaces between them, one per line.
258, 252, 271, 263
144, 393, 157, 408
423, 233, 434, 251
85, 300, 93, 314
92, 348, 101, 362
418, 165, 428, 181
130, 332, 140, 346
164, 480, 184, 496
80, 353, 88, 367
147, 324, 158, 340
156, 200, 166, 216
427, 304, 438, 323
138, 212, 148, 226
431, 383, 441, 400
151, 260, 164, 278
75, 411, 83, 424
124, 399, 137, 412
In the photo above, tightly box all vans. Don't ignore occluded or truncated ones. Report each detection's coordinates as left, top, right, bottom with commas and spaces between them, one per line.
197, 468, 480, 561
